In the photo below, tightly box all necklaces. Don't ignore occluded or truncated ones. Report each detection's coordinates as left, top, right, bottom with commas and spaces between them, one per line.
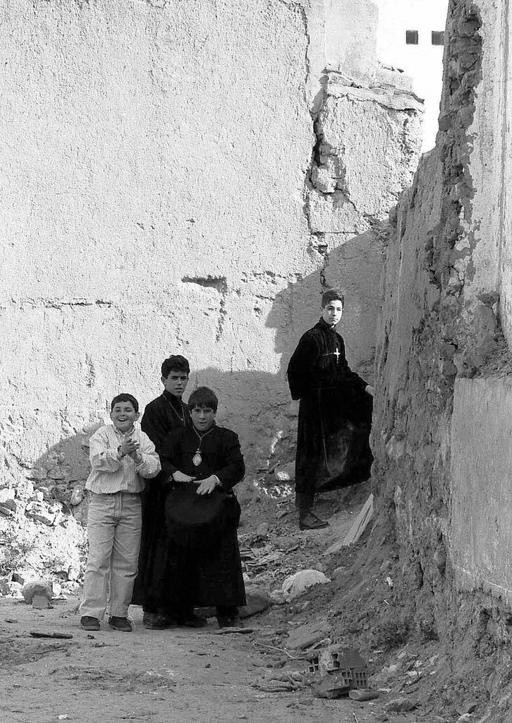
333, 346, 342, 365
162, 395, 187, 428
192, 426, 214, 467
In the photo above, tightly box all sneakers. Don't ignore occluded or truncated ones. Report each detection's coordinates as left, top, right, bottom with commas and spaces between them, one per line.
218, 609, 233, 627
109, 616, 132, 632
143, 612, 207, 630
81, 616, 100, 631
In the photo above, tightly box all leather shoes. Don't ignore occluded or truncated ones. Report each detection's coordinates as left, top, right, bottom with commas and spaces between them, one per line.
299, 513, 329, 530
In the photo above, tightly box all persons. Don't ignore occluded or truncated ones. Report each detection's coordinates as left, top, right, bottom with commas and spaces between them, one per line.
78, 392, 162, 632
159, 386, 248, 630
131, 355, 192, 630
287, 288, 375, 530
